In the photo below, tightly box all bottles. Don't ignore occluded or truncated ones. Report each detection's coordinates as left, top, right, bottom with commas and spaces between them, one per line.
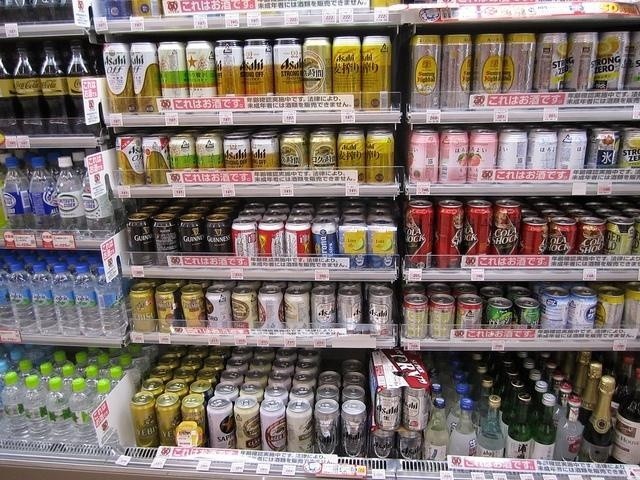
155, 391, 182, 445
66, 44, 97, 138
23, 375, 48, 445
51, 263, 83, 337
506, 393, 531, 459
618, 356, 635, 382
14, 47, 45, 136
109, 186, 129, 234
0, 344, 145, 379
85, 47, 105, 78
28, 154, 61, 232
99, 380, 120, 449
39, 45, 71, 135
6, 261, 36, 334
95, 266, 128, 340
1, 251, 105, 263
73, 264, 103, 340
259, 397, 288, 452
80, 155, 118, 240
564, 31, 598, 91
575, 350, 591, 394
556, 397, 583, 460
72, 152, 86, 176
68, 377, 98, 448
0, 372, 28, 442
596, 31, 628, 91
339, 223, 368, 254
223, 132, 250, 169
0, 271, 12, 333
529, 392, 557, 459
472, 34, 504, 96
428, 350, 572, 395
580, 376, 616, 463
205, 213, 231, 255
0, 57, 15, 136
581, 362, 603, 430
28, 263, 60, 338
422, 397, 448, 462
44, 155, 63, 181
503, 32, 536, 93
446, 399, 476, 456
411, 34, 442, 111
47, 375, 72, 446
613, 369, 640, 466
478, 396, 506, 457
55, 155, 87, 239
2, 156, 31, 230
624, 30, 640, 90
533, 33, 568, 94
442, 34, 472, 110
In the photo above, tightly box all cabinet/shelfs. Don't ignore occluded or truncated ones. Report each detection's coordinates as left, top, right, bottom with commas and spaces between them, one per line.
101, 0, 427, 480
0, 0, 119, 480
404, 0, 640, 480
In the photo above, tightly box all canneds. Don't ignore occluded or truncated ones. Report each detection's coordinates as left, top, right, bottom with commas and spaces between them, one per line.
153, 212, 178, 253
436, 200, 465, 268
397, 433, 424, 461
541, 287, 569, 330
128, 282, 156, 334
438, 128, 467, 185
130, 393, 160, 450
125, 213, 155, 252
196, 133, 222, 169
186, 40, 218, 97
452, 283, 478, 295
427, 283, 452, 294
279, 130, 308, 169
497, 129, 527, 171
233, 395, 260, 450
522, 217, 550, 256
568, 285, 595, 330
369, 285, 394, 334
402, 293, 429, 341
205, 283, 230, 331
341, 400, 367, 456
515, 296, 540, 330
577, 215, 605, 254
157, 41, 189, 99
274, 37, 303, 96
480, 286, 504, 297
250, 131, 279, 171
405, 128, 439, 185
485, 297, 513, 328
314, 399, 341, 453
403, 387, 429, 432
594, 285, 624, 330
238, 201, 395, 217
311, 286, 336, 330
138, 201, 236, 211
362, 34, 392, 110
494, 200, 521, 256
404, 283, 425, 293
304, 36, 332, 96
506, 285, 532, 297
311, 217, 339, 256
130, 41, 160, 114
178, 213, 204, 251
367, 218, 397, 255
180, 284, 206, 328
104, 42, 136, 114
404, 201, 435, 270
285, 217, 312, 258
167, 134, 197, 169
215, 39, 244, 96
621, 128, 640, 171
375, 387, 403, 431
140, 347, 368, 401
309, 131, 337, 168
525, 197, 640, 220
286, 399, 314, 452
455, 294, 483, 330
588, 128, 621, 169
465, 200, 493, 257
366, 131, 395, 185
428, 295, 456, 341
332, 36, 362, 109
180, 393, 207, 449
141, 134, 168, 187
338, 132, 366, 184
155, 283, 182, 333
625, 283, 640, 329
242, 39, 274, 98
558, 128, 588, 171
257, 216, 286, 258
549, 217, 577, 254
527, 128, 557, 169
467, 128, 498, 184
208, 395, 234, 448
284, 284, 311, 332
231, 217, 258, 258
337, 287, 362, 328
605, 215, 635, 255
370, 427, 397, 458
115, 134, 146, 187
231, 284, 257, 332
260, 284, 284, 333
